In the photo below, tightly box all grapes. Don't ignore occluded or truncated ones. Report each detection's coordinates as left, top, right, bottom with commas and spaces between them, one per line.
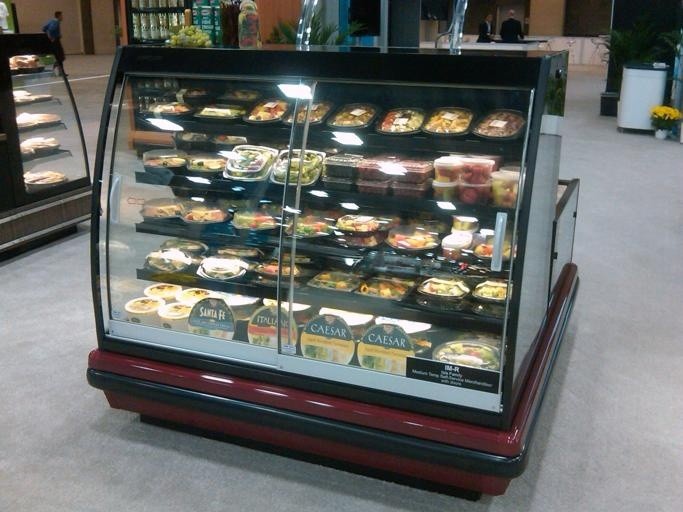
170, 25, 212, 47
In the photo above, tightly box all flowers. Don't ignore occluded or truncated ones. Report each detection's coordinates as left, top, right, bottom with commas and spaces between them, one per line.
647, 105, 681, 132
164, 24, 212, 49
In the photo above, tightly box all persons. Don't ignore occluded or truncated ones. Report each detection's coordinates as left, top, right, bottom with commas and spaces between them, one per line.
41, 11, 68, 77
499, 8, 523, 43
476, 12, 494, 43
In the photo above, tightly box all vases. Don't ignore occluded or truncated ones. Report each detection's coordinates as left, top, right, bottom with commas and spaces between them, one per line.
653, 128, 670, 139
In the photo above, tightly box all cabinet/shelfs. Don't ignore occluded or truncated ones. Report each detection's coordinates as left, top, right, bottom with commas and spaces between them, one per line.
80, 41, 581, 501
116, 1, 240, 157
0, 31, 102, 259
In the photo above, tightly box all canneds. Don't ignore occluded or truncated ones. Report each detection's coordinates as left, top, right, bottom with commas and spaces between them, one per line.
131, 0, 185, 40
191, 0, 223, 47
